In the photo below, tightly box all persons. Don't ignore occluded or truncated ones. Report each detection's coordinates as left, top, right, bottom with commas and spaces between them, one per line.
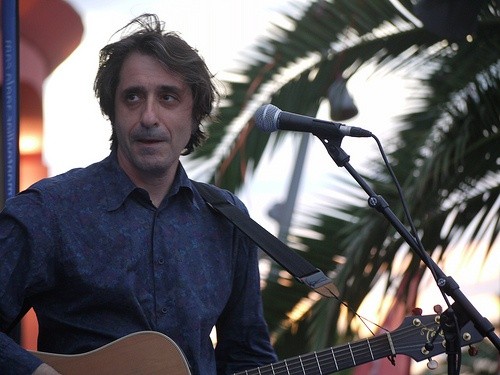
0, 12, 279, 374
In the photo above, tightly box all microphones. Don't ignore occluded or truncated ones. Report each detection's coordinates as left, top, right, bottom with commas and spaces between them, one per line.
254, 103, 372, 139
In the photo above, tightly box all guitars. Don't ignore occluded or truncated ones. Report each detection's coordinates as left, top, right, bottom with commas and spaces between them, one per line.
18, 300, 489, 375
